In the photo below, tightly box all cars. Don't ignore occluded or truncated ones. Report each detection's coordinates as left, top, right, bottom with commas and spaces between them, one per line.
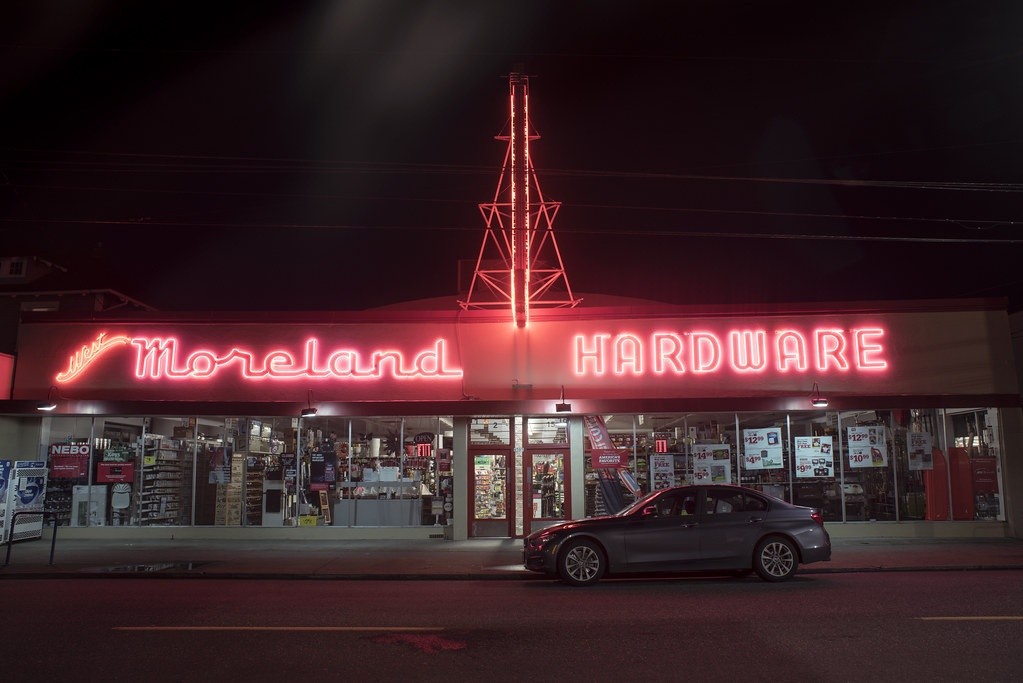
520, 484, 832, 586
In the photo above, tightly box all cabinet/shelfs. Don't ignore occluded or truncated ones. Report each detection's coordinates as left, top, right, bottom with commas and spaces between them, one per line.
133, 437, 215, 526
216, 450, 264, 527
473, 456, 505, 520
338, 456, 435, 514
